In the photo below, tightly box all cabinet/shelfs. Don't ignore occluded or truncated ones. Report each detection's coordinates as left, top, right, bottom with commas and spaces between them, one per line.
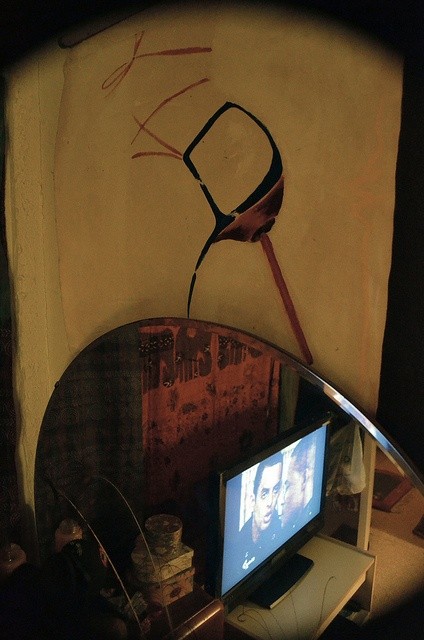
116, 326, 281, 594
121, 582, 224, 640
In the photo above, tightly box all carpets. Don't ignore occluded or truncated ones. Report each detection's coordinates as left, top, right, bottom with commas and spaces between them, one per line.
372, 470, 414, 513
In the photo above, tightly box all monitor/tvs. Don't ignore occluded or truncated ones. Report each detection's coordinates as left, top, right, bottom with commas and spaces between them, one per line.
215, 410, 331, 611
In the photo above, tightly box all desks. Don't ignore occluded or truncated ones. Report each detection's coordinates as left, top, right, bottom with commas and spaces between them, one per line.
225, 532, 378, 639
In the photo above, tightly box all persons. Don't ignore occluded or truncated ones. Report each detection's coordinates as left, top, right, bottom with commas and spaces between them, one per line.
237, 451, 285, 583
281, 438, 318, 543
